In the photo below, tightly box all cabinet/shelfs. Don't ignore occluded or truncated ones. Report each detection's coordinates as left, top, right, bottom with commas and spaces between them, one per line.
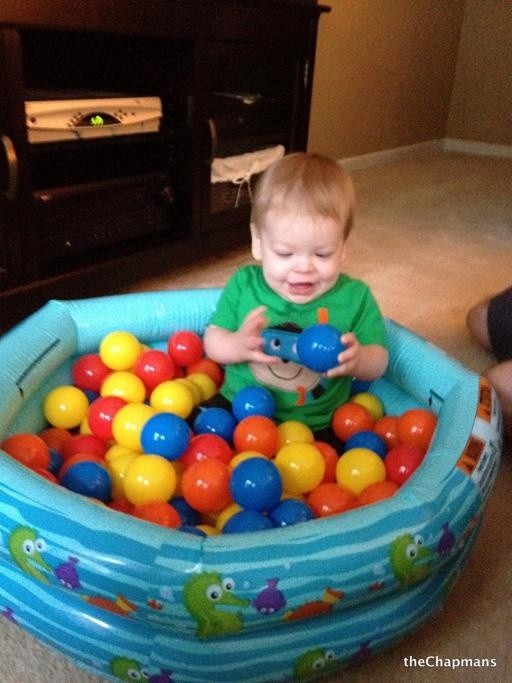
0, 0, 328, 330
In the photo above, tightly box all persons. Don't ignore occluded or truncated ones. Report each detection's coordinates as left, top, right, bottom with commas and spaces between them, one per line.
466, 286, 512, 432
184, 153, 389, 458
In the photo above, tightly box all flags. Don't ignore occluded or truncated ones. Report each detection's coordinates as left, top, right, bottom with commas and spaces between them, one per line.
194, 407, 237, 448
175, 378, 202, 406
45, 449, 65, 478
58, 453, 105, 483
332, 402, 372, 443
123, 454, 176, 506
280, 490, 308, 505
1, 433, 50, 471
386, 450, 425, 487
39, 429, 73, 453
141, 414, 189, 461
196, 525, 222, 537
350, 395, 385, 421
307, 483, 360, 521
82, 390, 101, 404
105, 444, 142, 500
358, 481, 400, 506
222, 511, 274, 537
180, 434, 231, 469
102, 371, 145, 405
273, 421, 316, 459
312, 441, 340, 483
230, 458, 284, 512
345, 431, 388, 464
29, 467, 60, 486
268, 498, 317, 529
169, 332, 205, 368
59, 436, 110, 461
81, 416, 95, 436
229, 452, 269, 469
139, 342, 151, 355
101, 331, 141, 370
198, 513, 219, 528
187, 373, 216, 401
234, 415, 282, 460
182, 459, 232, 513
373, 415, 404, 451
217, 504, 244, 530
109, 498, 135, 515
75, 355, 114, 393
400, 410, 439, 451
135, 351, 176, 387
61, 462, 111, 505
176, 525, 206, 537
130, 500, 182, 532
146, 387, 153, 399
44, 386, 90, 430
336, 448, 386, 499
150, 380, 194, 420
299, 326, 347, 374
112, 403, 157, 450
88, 397, 128, 440
272, 444, 327, 494
167, 496, 201, 527
348, 378, 373, 397
233, 385, 277, 421
187, 359, 223, 392
170, 460, 186, 498
